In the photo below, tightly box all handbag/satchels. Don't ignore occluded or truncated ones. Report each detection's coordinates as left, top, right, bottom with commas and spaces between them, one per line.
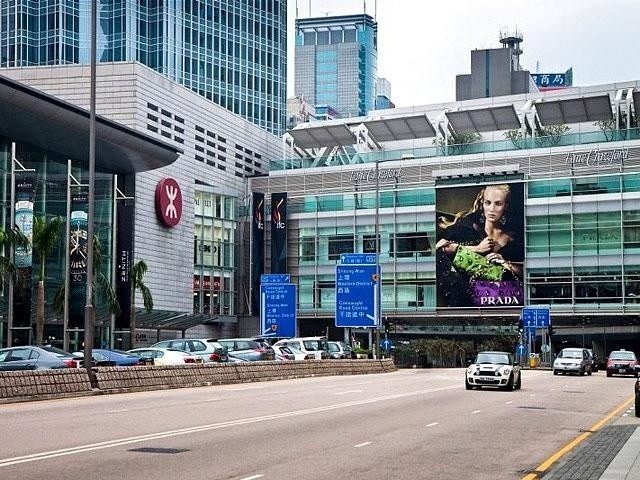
453, 245, 507, 281
471, 281, 524, 306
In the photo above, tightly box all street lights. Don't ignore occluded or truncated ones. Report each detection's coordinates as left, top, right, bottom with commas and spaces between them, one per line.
374, 153, 414, 359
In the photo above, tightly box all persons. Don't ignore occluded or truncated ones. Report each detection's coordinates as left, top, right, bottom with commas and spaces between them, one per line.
436, 185, 523, 279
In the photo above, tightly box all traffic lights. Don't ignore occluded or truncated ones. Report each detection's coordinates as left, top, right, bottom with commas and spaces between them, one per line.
518, 320, 524, 333
550, 326, 557, 337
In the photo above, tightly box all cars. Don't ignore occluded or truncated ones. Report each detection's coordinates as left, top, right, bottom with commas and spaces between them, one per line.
635, 364, 640, 418
604, 349, 639, 378
70, 350, 117, 368
125, 347, 205, 366
0, 345, 97, 372
586, 349, 599, 373
464, 351, 522, 391
552, 346, 593, 377
149, 337, 354, 363
80, 348, 155, 366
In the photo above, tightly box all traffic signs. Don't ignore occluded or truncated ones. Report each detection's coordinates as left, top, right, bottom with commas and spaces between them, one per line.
522, 308, 550, 328
261, 274, 297, 337
335, 253, 381, 328
